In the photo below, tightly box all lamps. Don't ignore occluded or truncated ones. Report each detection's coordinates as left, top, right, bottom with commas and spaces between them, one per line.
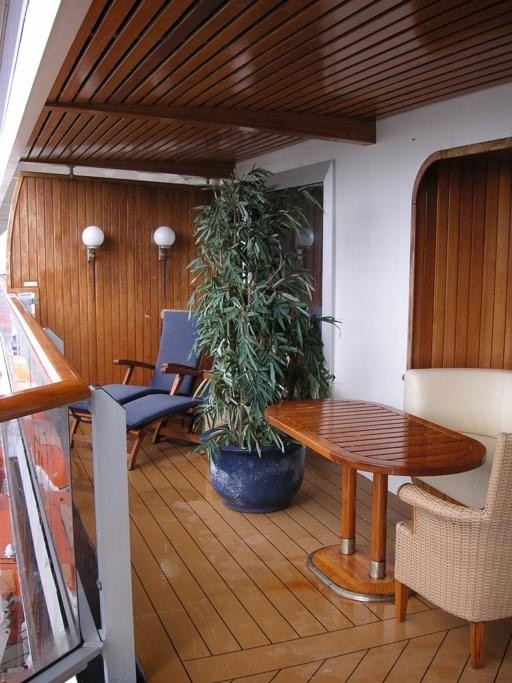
154, 225, 176, 262
82, 225, 104, 262
293, 227, 314, 271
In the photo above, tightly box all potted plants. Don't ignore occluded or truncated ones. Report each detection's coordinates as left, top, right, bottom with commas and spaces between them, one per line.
186, 161, 343, 514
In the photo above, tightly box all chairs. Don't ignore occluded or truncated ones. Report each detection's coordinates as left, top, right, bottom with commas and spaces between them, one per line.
394, 368, 512, 668
70, 305, 216, 471
